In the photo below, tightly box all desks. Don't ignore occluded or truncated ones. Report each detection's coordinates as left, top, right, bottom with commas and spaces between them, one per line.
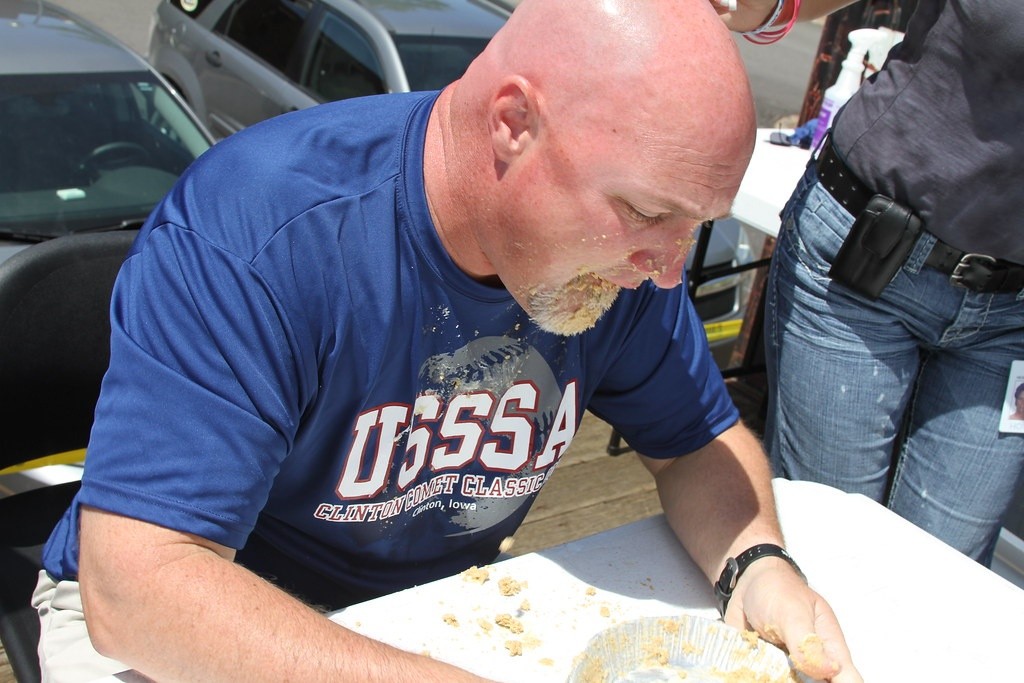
51, 476, 1024, 683
606, 128, 821, 457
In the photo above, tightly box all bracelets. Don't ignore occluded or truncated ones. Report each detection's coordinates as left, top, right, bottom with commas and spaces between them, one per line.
714, 543, 808, 619
741, 0, 801, 45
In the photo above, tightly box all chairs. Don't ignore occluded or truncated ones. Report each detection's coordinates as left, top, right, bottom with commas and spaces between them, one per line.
0, 235, 136, 683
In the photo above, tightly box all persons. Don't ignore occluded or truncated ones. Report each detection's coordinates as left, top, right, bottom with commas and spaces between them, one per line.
707, 0, 1024, 566
31, 0, 864, 683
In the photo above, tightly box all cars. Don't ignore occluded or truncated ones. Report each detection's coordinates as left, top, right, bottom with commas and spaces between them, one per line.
143, 0, 758, 351
0, 0, 221, 271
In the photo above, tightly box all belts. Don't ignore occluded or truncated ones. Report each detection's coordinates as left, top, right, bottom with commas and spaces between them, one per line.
816, 133, 1024, 293
